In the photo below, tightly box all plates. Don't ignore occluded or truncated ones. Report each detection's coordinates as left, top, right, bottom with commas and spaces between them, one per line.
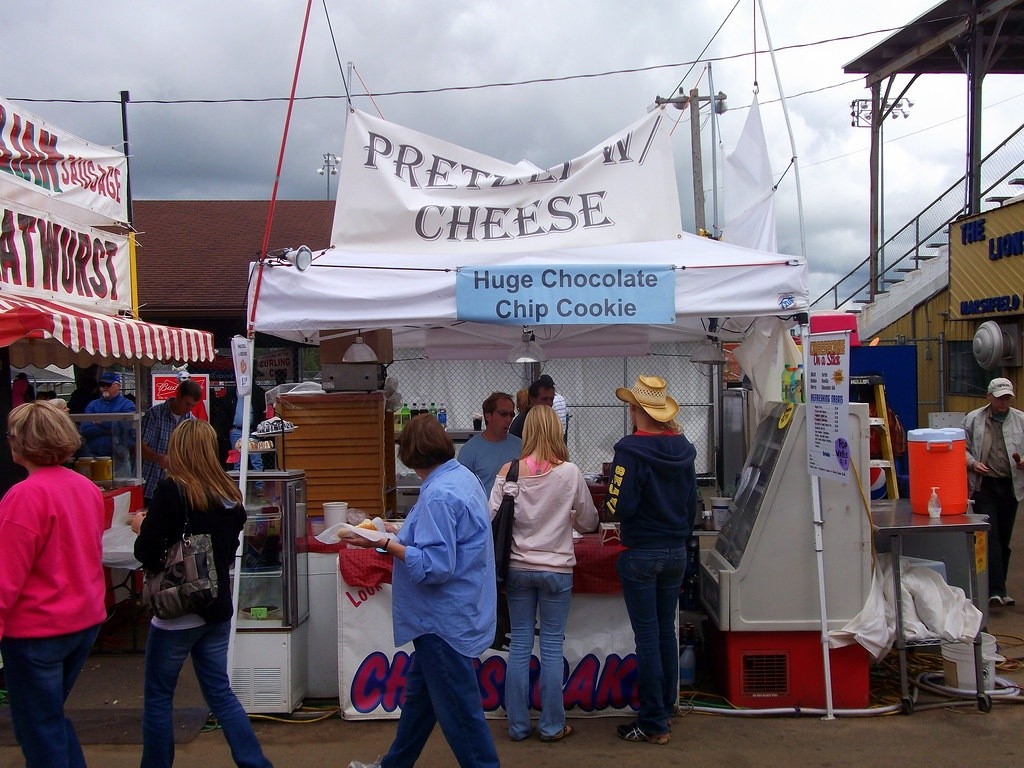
239, 607, 280, 617
239, 448, 276, 454
251, 426, 298, 435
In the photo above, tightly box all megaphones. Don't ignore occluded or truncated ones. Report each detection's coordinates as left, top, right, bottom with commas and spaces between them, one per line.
672, 87, 690, 109
278, 245, 313, 273
714, 91, 727, 114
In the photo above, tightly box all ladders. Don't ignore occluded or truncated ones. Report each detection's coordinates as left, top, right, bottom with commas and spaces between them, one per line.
849, 375, 899, 500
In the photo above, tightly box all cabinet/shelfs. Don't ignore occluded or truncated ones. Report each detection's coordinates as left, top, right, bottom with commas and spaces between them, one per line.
228, 471, 309, 629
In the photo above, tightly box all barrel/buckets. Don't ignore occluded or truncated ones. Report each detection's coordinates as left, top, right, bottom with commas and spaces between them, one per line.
908, 428, 969, 516
940, 632, 1005, 700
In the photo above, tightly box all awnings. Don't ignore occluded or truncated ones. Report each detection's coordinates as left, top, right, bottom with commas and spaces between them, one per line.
0, 292, 217, 364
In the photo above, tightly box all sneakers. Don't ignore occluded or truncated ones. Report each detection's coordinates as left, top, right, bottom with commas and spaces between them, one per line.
616, 721, 670, 744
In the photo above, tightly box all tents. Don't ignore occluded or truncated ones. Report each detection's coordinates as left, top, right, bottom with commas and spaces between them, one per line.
226, 231, 836, 722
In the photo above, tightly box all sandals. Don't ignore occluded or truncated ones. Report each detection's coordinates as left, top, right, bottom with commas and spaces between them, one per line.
542, 724, 575, 741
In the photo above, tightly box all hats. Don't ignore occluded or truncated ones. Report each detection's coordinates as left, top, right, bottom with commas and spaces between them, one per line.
616, 375, 680, 423
856, 374, 885, 392
16, 373, 27, 378
987, 378, 1016, 398
87, 380, 99, 391
540, 375, 555, 385
125, 393, 136, 405
99, 372, 120, 383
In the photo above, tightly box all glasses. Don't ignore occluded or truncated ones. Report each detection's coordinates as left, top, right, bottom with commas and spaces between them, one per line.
992, 394, 1013, 400
179, 377, 190, 379
497, 409, 515, 418
99, 381, 112, 387
4, 432, 15, 440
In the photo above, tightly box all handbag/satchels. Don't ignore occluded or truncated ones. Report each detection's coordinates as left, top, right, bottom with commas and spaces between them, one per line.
133, 478, 218, 619
887, 409, 907, 456
491, 459, 520, 580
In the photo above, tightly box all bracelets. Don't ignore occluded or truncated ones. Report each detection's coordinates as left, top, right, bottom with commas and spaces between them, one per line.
383, 538, 390, 550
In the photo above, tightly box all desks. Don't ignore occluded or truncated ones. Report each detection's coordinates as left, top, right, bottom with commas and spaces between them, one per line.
871, 501, 994, 714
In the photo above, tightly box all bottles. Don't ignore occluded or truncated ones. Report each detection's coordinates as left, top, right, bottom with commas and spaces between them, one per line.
420, 403, 428, 414
781, 365, 806, 403
437, 401, 447, 431
94, 456, 113, 480
411, 403, 420, 419
693, 485, 733, 530
428, 403, 437, 419
311, 517, 325, 536
401, 402, 411, 429
680, 622, 701, 687
75, 457, 95, 481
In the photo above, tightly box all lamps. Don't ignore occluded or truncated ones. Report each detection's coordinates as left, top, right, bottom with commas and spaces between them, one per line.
689, 318, 727, 363
502, 325, 550, 363
342, 328, 379, 361
257, 245, 315, 272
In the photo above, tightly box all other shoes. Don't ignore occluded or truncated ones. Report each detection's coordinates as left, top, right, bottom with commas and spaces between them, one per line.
348, 755, 382, 768
989, 595, 1003, 607
1002, 596, 1015, 606
256, 488, 264, 497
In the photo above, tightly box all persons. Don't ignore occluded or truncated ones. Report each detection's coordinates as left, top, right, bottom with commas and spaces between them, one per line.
141, 380, 202, 509
488, 404, 599, 742
11, 373, 58, 409
342, 412, 501, 768
603, 373, 697, 746
131, 418, 273, 768
457, 392, 522, 501
0, 400, 107, 768
960, 378, 1024, 608
210, 366, 266, 497
509, 375, 569, 448
47, 363, 136, 477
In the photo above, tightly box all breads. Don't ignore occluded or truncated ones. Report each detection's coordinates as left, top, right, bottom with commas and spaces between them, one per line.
336, 520, 378, 538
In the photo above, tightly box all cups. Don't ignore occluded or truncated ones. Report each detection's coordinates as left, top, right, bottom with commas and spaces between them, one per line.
394, 412, 402, 431
473, 415, 483, 431
322, 502, 348, 528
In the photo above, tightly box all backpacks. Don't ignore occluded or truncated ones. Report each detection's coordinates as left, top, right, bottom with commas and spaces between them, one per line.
67, 389, 91, 412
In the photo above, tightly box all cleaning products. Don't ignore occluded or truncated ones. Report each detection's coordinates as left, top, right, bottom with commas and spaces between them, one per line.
928, 487, 942, 518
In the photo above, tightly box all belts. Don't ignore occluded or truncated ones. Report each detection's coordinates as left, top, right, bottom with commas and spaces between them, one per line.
232, 425, 253, 430
